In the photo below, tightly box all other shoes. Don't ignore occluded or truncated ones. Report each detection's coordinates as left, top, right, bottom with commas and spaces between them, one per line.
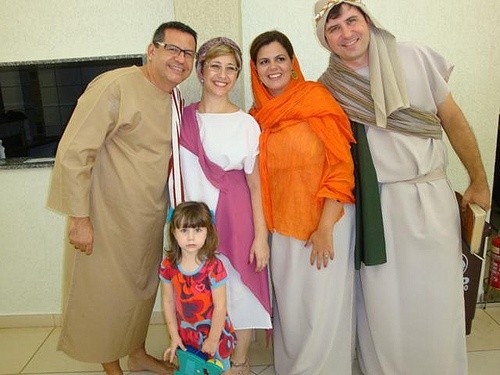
230, 357, 251, 375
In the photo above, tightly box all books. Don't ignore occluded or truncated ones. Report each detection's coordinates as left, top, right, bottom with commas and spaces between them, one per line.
454, 191, 487, 254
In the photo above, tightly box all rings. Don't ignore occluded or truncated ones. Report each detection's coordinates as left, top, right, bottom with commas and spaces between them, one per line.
323, 255, 329, 258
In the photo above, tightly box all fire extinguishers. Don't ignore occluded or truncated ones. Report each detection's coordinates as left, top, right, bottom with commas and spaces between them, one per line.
489, 229, 500, 290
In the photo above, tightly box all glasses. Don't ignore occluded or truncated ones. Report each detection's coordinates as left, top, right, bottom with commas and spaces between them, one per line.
155, 41, 196, 60
203, 61, 240, 74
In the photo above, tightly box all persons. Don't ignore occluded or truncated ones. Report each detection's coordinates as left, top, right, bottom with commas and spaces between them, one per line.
248, 30, 357, 375
159, 201, 237, 375
48, 22, 197, 375
178, 36, 269, 375
315, 0, 491, 375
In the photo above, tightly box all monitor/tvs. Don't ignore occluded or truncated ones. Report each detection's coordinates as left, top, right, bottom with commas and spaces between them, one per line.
0, 53, 148, 169
